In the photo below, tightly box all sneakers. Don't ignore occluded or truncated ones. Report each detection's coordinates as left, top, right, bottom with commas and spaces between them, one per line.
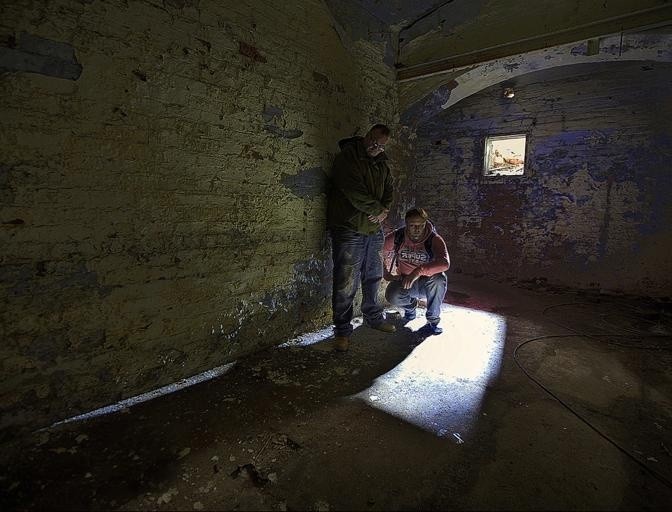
427, 317, 445, 334
405, 308, 417, 321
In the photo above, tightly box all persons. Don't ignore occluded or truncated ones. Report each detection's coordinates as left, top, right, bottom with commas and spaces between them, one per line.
381, 205, 450, 335
326, 123, 395, 353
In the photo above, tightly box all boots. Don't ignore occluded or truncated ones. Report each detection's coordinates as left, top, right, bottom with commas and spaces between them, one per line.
361, 314, 397, 333
334, 332, 350, 351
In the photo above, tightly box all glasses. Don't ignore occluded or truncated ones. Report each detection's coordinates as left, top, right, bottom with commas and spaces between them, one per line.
374, 143, 385, 153
